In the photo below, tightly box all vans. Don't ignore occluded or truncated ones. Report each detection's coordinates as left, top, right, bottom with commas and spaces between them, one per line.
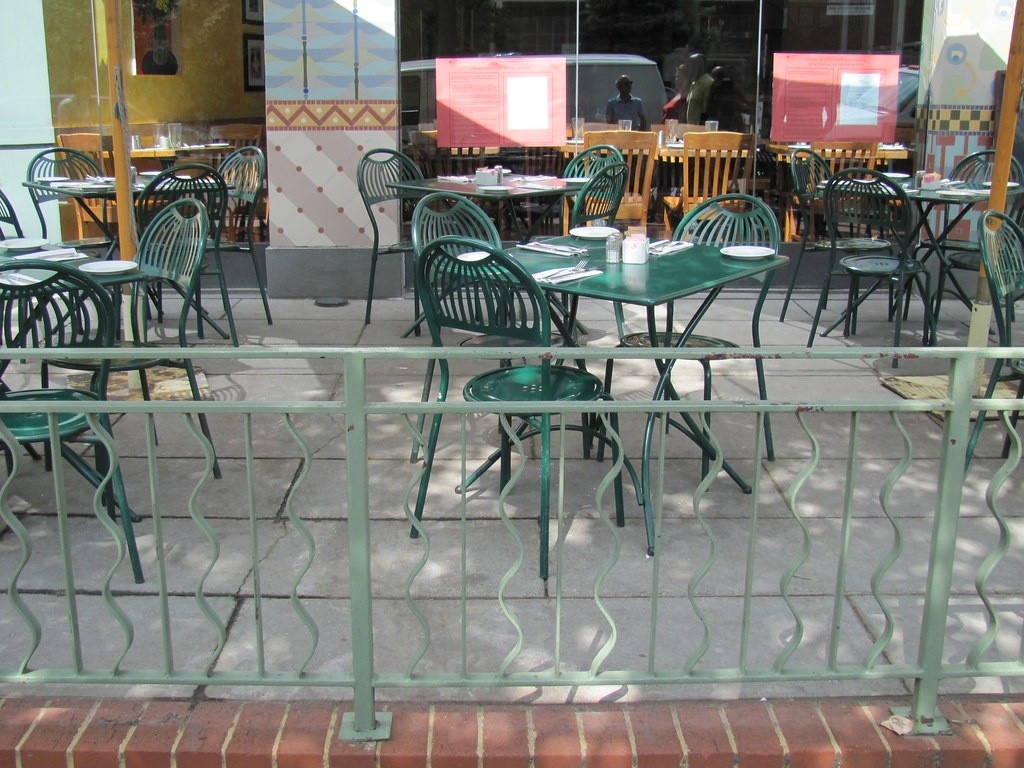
400, 54, 669, 137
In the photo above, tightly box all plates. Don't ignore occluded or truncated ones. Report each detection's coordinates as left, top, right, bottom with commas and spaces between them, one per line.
982, 181, 1020, 187
36, 177, 70, 182
502, 169, 512, 176
720, 246, 776, 261
560, 177, 592, 186
667, 144, 683, 148
0, 238, 50, 252
882, 173, 910, 178
569, 227, 621, 241
205, 143, 230, 147
457, 252, 514, 266
935, 190, 974, 198
478, 185, 514, 194
78, 260, 137, 276
139, 171, 161, 176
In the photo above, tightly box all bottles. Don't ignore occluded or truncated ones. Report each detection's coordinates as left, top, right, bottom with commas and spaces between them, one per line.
611, 232, 623, 261
131, 135, 141, 149
494, 165, 503, 185
130, 166, 137, 185
605, 235, 621, 264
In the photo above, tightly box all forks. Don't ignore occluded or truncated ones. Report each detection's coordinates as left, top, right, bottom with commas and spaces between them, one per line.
543, 260, 588, 279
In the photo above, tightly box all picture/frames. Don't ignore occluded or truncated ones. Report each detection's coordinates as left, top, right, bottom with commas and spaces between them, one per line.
242, 33, 265, 93
242, 0, 258, 26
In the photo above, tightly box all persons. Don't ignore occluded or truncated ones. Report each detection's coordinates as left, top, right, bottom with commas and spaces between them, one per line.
662, 64, 690, 125
686, 53, 743, 134
57, 77, 92, 124
606, 74, 646, 132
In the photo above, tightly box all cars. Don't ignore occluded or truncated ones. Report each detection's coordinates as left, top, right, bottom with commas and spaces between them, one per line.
840, 64, 920, 125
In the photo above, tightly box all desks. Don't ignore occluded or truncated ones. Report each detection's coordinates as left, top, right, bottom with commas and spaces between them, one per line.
96, 146, 236, 202
812, 178, 1024, 346
0, 244, 157, 523
21, 175, 243, 341
553, 139, 748, 223
382, 175, 608, 339
440, 235, 791, 557
765, 143, 909, 240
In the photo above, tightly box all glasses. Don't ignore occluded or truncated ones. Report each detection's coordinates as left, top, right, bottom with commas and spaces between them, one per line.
620, 81, 633, 85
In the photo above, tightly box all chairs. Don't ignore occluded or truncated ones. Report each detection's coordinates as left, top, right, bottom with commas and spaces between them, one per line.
0, 122, 1024, 585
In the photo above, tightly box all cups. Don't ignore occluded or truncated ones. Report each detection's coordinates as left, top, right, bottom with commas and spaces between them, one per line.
618, 119, 632, 130
151, 123, 166, 148
571, 117, 585, 142
168, 123, 182, 150
705, 121, 719, 132
664, 118, 679, 143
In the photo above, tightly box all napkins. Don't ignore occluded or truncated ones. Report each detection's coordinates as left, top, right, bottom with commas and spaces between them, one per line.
531, 267, 604, 285
509, 174, 558, 183
437, 175, 477, 184
515, 241, 588, 257
86, 176, 116, 182
134, 180, 146, 189
13, 247, 90, 262
944, 180, 966, 187
889, 188, 920, 195
518, 182, 565, 190
649, 239, 694, 256
49, 182, 95, 189
